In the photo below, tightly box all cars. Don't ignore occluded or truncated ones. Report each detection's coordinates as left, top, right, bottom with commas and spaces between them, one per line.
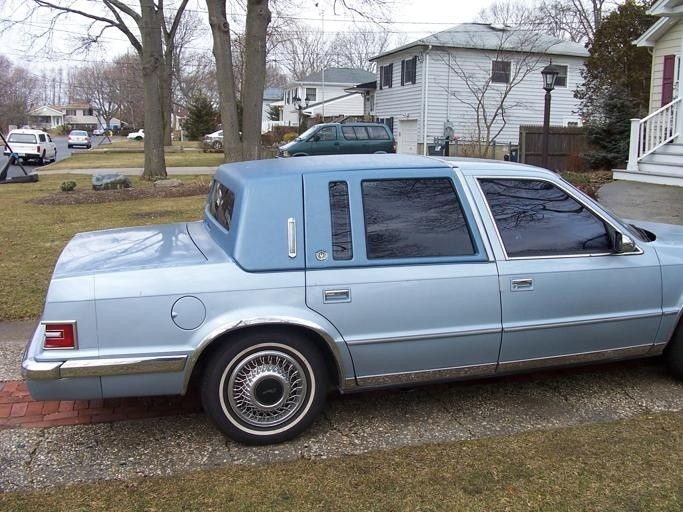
203, 129, 242, 150
92, 124, 120, 136
66, 130, 92, 149
21, 154, 683, 448
126, 128, 144, 141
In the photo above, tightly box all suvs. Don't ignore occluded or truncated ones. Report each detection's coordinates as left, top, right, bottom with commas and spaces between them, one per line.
3, 129, 57, 166
274, 123, 398, 158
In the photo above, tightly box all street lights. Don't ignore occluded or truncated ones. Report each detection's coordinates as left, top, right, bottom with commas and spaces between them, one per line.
539, 58, 561, 169
292, 95, 310, 135
